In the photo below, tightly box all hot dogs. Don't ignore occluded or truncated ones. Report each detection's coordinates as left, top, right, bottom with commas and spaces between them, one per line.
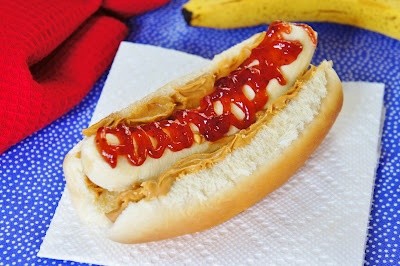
62, 20, 344, 244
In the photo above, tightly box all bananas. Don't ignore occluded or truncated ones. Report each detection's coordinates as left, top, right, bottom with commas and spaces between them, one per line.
181, 0, 400, 41
